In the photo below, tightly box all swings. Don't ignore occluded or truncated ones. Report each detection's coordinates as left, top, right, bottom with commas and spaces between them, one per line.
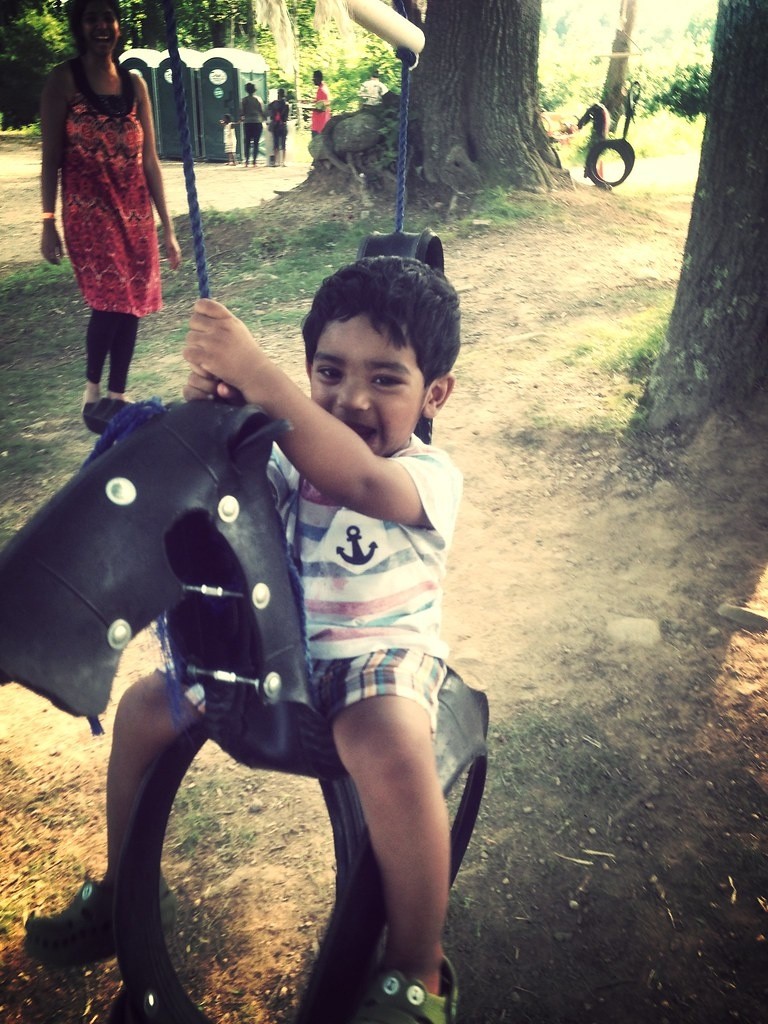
0, 0, 491, 1023
577, 0, 646, 190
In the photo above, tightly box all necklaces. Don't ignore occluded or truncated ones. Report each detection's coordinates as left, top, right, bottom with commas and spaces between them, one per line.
69, 58, 134, 117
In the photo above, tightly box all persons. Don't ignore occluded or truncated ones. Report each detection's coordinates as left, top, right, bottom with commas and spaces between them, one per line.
218, 114, 245, 166
240, 82, 267, 167
40, 0, 181, 409
301, 70, 331, 170
360, 73, 388, 107
560, 123, 576, 133
266, 89, 289, 167
28, 256, 463, 1024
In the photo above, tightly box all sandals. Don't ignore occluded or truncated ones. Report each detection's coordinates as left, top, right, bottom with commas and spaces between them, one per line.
23, 869, 182, 967
350, 954, 461, 1024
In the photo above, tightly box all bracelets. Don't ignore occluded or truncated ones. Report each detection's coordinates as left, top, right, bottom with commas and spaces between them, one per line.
42, 213, 54, 218
